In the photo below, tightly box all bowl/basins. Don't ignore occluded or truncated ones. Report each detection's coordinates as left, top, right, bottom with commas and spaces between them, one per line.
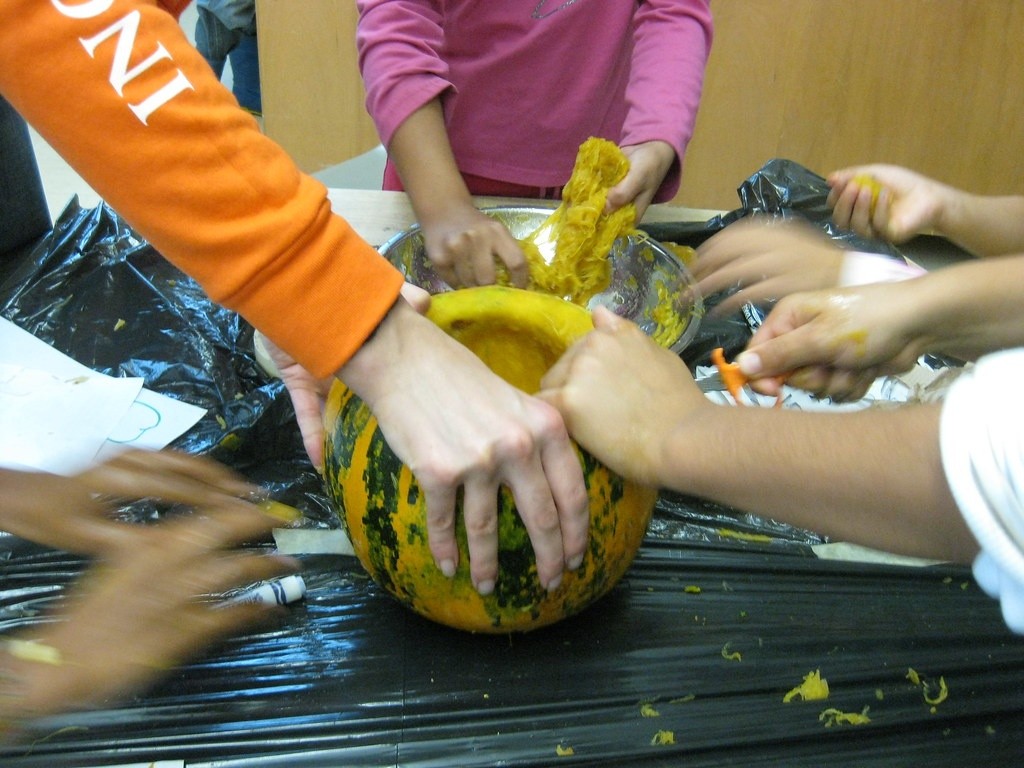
376, 205, 704, 354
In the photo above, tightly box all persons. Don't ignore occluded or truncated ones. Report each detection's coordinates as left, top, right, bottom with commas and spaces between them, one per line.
685, 159, 1023, 316
535, 252, 1023, 636
158, 0, 261, 120
0, 0, 595, 592
0, 444, 308, 726
357, 2, 715, 290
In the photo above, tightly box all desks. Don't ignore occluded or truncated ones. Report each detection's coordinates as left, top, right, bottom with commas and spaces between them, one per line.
0, 184, 1024, 768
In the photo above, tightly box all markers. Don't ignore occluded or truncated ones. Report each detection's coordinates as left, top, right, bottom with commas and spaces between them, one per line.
208, 574, 309, 609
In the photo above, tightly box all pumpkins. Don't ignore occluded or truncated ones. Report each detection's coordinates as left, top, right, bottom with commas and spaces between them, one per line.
319, 285, 656, 635
